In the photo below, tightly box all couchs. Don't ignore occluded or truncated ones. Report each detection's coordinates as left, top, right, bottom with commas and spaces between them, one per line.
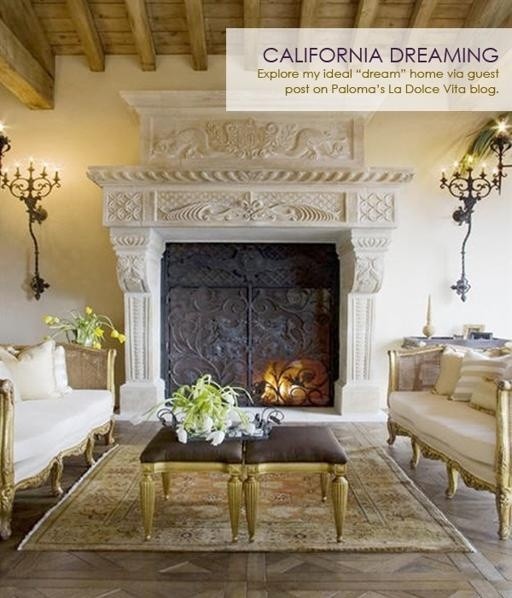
386, 346, 511, 540
0, 339, 118, 539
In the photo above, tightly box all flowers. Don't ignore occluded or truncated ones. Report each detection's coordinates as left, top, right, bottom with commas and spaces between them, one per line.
138, 374, 266, 448
45, 304, 126, 351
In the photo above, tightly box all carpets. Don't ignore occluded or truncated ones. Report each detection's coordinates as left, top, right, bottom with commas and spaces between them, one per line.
18, 441, 476, 550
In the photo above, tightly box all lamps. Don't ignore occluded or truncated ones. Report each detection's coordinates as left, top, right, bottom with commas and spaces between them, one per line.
440, 118, 511, 302
0, 120, 63, 300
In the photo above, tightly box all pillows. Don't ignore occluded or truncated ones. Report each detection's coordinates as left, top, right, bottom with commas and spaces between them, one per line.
430, 347, 512, 417
1, 340, 72, 401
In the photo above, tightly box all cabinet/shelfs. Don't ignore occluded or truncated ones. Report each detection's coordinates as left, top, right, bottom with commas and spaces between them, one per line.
403, 336, 511, 350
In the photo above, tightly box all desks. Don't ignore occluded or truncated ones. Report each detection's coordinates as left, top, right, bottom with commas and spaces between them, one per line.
141, 427, 348, 543
